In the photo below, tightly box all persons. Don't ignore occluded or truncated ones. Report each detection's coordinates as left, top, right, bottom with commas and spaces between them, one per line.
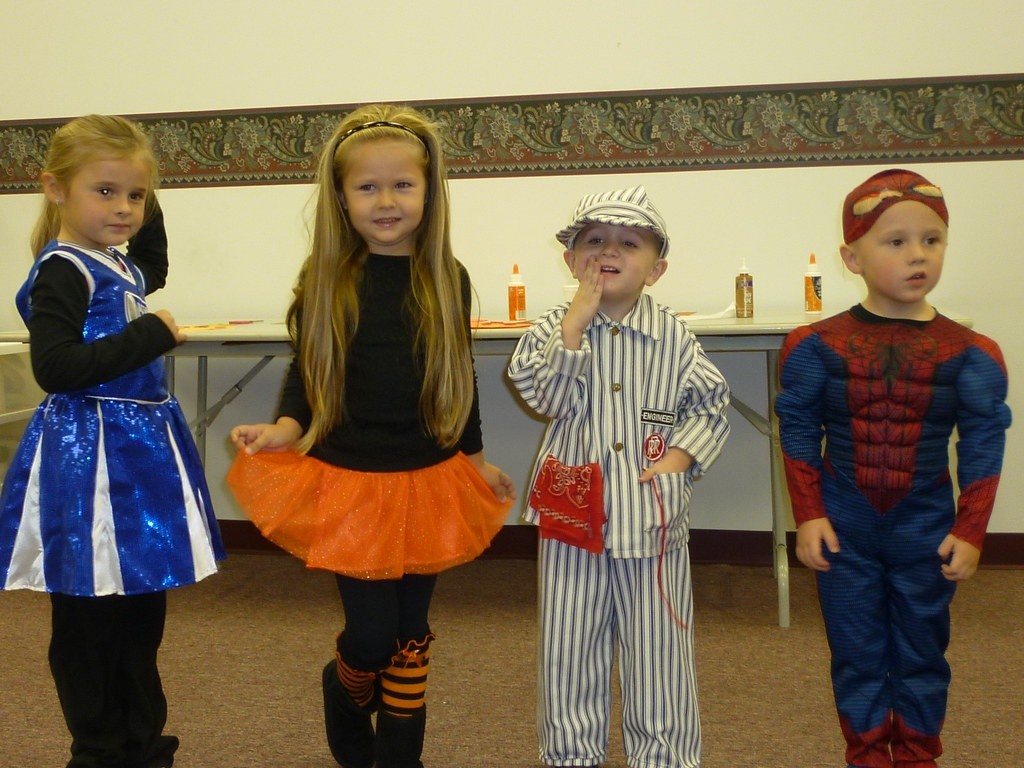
218, 99, 519, 768
0, 112, 230, 765
502, 180, 731, 768
772, 166, 1012, 768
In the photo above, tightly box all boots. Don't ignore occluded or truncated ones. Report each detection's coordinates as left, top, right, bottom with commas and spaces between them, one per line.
374, 703, 429, 768
323, 659, 381, 767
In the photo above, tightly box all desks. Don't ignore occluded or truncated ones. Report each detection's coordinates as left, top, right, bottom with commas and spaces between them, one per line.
2, 315, 979, 628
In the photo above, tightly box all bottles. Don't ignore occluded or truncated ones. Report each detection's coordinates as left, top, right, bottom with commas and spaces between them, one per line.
507, 264, 526, 322
804, 251, 822, 314
736, 266, 754, 318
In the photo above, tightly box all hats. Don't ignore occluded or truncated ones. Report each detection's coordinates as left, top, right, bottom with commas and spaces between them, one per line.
555, 186, 671, 262
843, 169, 950, 244
